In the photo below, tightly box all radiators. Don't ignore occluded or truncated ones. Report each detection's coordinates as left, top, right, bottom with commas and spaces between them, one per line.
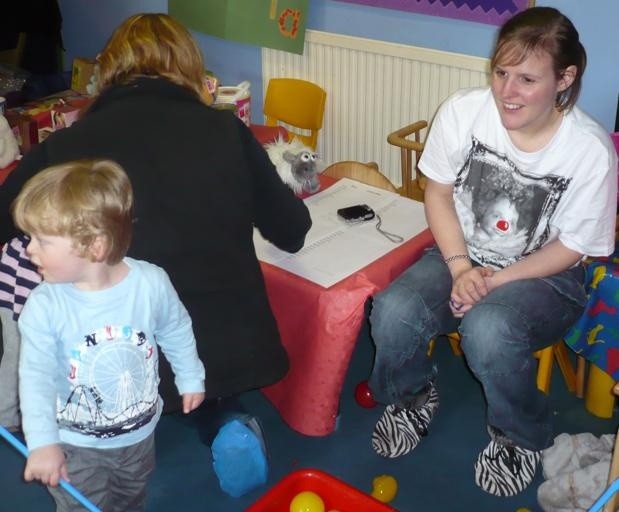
260, 28, 493, 190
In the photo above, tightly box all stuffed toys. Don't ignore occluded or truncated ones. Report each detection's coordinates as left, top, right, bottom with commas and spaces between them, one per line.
265, 132, 323, 194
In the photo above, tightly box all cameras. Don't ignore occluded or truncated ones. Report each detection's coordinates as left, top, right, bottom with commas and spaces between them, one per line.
337, 204, 375, 226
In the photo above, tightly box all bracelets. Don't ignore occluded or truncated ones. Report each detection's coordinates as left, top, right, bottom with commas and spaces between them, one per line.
443, 256, 473, 265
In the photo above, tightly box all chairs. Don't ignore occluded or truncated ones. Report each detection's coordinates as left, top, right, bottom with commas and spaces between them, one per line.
263, 78, 328, 173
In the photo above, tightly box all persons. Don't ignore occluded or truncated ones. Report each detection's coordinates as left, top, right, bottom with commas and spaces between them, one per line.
0, 236, 44, 431
9, 157, 208, 512
370, 5, 616, 497
0, 13, 312, 498
